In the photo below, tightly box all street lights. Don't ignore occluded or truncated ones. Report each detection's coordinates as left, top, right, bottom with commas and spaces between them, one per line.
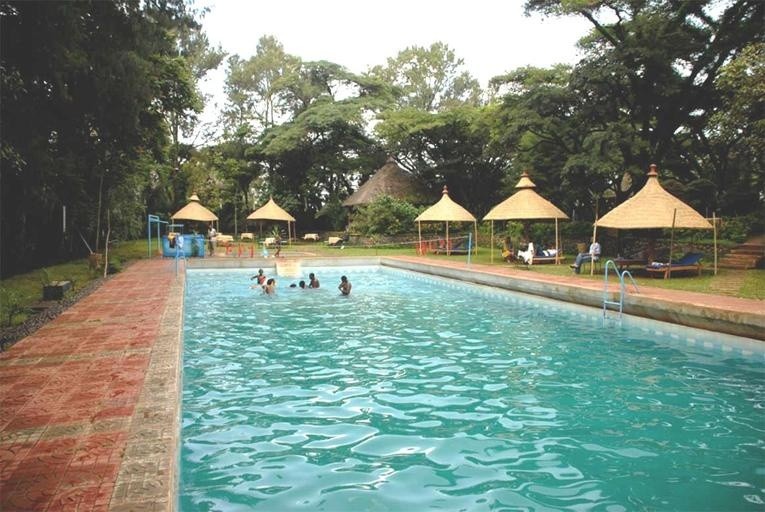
502, 250, 511, 257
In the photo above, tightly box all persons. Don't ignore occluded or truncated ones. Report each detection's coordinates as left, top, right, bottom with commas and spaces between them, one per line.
308, 272, 320, 289
338, 275, 352, 297
517, 240, 538, 265
504, 236, 520, 264
249, 269, 266, 290
299, 281, 306, 289
261, 278, 275, 296
206, 224, 217, 257
647, 244, 695, 268
569, 234, 601, 274
273, 232, 282, 257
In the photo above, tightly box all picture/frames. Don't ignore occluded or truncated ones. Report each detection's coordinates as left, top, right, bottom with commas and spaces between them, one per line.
614, 260, 648, 275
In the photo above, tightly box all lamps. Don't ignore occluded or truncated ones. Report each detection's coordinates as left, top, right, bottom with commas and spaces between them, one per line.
430, 247, 604, 275
646, 252, 705, 280
238, 232, 254, 258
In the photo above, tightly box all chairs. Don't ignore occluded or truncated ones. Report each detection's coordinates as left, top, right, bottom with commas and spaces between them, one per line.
569, 263, 579, 269
